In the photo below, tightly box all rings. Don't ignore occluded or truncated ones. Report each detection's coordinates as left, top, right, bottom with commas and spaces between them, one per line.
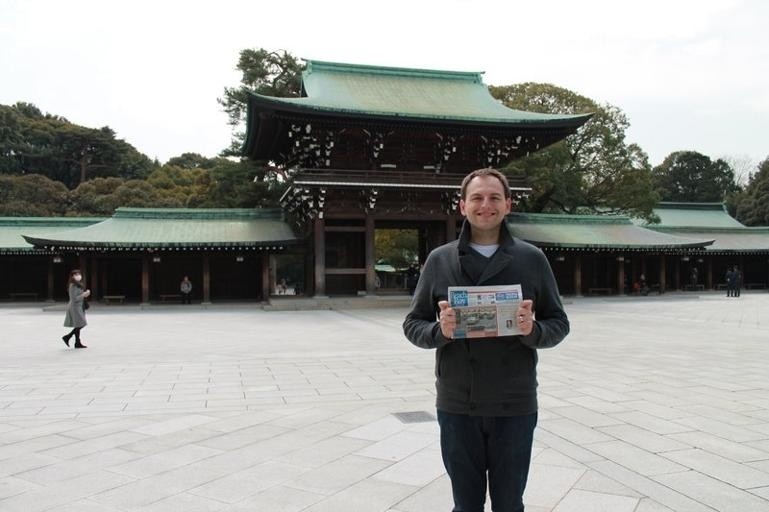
519, 317, 523, 322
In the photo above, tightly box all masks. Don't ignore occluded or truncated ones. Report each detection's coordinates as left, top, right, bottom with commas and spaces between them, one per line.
74, 275, 82, 282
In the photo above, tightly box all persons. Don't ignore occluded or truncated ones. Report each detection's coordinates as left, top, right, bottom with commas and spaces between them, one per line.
691, 266, 698, 291
179, 275, 192, 304
401, 167, 570, 512
61, 269, 90, 348
723, 267, 736, 297
407, 263, 424, 296
732, 264, 744, 297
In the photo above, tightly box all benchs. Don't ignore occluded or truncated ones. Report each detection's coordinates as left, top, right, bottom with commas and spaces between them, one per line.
714, 284, 728, 290
160, 294, 192, 303
10, 293, 39, 301
589, 287, 613, 296
630, 282, 661, 295
104, 296, 126, 305
681, 284, 705, 291
745, 283, 767, 290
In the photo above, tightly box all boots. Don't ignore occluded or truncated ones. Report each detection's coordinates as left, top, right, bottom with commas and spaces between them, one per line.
62, 333, 72, 347
75, 339, 86, 348
